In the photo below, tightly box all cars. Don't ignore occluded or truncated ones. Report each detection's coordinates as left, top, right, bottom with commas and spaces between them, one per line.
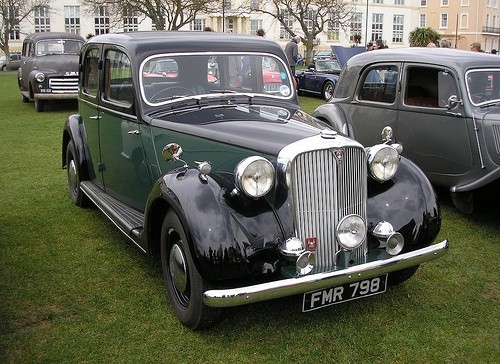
291, 44, 385, 103
139, 56, 299, 97
61, 30, 449, 333
0, 53, 22, 72
16, 31, 86, 113
308, 46, 500, 216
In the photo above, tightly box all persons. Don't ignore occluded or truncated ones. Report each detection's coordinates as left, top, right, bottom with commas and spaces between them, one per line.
491, 49, 497, 55
427, 42, 436, 48
376, 40, 388, 49
284, 36, 300, 77
367, 42, 375, 51
470, 42, 486, 53
439, 37, 450, 48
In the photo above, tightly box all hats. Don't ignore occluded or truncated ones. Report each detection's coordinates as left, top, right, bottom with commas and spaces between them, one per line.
367, 43, 373, 46
491, 49, 498, 52
